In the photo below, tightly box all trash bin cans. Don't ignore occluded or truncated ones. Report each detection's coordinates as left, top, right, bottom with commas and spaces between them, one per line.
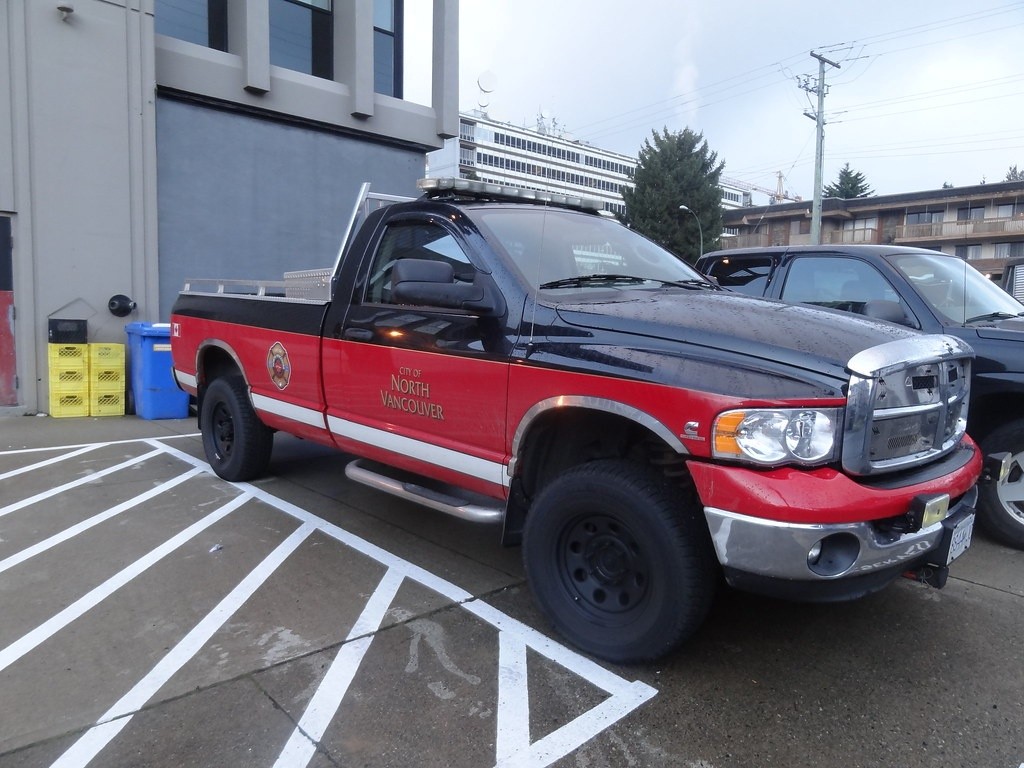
123, 320, 189, 420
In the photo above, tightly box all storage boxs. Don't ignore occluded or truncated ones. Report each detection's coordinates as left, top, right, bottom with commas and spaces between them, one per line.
48, 319, 125, 418
283, 267, 333, 301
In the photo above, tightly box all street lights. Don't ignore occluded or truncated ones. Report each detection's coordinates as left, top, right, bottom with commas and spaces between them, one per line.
679, 205, 703, 256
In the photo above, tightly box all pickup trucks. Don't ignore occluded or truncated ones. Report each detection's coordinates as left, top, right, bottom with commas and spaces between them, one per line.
170, 177, 982, 663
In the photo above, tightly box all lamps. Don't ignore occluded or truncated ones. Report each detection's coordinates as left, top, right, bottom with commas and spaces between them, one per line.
57, 0, 73, 21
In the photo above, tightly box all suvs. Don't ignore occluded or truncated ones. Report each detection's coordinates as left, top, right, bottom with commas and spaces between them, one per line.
696, 244, 1024, 548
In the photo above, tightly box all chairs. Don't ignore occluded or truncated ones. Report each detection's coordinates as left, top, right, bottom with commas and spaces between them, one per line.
835, 280, 877, 314
